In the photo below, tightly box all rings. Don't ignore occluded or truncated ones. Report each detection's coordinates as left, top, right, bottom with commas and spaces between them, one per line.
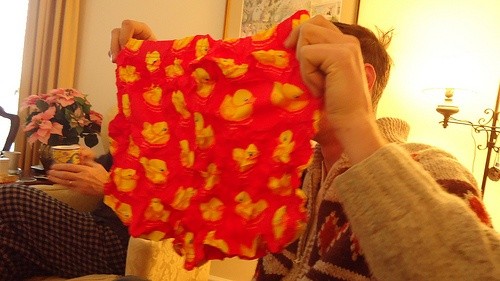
70, 180, 72, 185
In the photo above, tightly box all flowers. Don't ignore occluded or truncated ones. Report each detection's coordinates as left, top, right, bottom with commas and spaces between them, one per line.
19, 87, 104, 149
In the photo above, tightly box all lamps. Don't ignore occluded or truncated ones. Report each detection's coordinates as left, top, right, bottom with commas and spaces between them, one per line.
435, 84, 500, 199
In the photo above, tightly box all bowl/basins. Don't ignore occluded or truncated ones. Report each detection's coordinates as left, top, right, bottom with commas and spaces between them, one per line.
51, 146, 90, 164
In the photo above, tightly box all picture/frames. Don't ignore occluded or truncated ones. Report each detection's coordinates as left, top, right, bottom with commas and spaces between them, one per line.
223, 0, 360, 41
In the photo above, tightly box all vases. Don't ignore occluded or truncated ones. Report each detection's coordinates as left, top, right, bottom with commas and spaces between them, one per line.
39, 143, 82, 175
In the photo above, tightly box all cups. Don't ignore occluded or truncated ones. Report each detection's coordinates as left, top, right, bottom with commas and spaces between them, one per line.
0, 158, 10, 177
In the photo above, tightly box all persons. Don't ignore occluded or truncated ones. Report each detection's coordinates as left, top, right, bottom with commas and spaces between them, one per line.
0, 150, 130, 280
107, 14, 499, 280
